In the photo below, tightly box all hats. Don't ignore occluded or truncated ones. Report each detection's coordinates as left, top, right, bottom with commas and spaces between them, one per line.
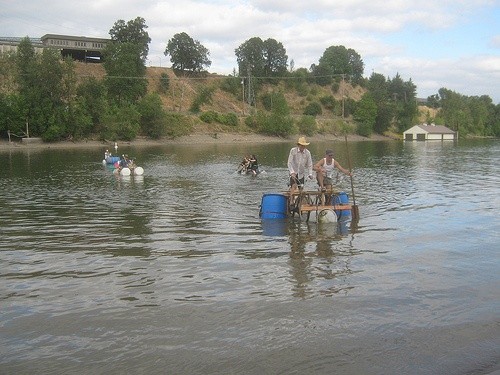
296, 136, 311, 146
326, 150, 335, 157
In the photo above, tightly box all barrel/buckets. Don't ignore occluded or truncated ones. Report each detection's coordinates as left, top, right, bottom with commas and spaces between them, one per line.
261, 194, 288, 218
332, 192, 350, 215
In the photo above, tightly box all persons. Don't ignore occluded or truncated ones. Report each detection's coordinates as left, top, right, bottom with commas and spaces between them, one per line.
235, 154, 259, 176
104, 149, 134, 170
287, 137, 314, 210
312, 149, 352, 204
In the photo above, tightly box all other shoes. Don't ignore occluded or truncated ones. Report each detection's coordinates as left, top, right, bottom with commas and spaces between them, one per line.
290, 201, 295, 206
321, 187, 326, 192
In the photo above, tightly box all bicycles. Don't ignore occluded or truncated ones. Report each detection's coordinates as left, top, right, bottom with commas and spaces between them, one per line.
287, 175, 313, 222
315, 171, 342, 221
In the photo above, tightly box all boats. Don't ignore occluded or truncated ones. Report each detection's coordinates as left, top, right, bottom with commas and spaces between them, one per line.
112, 160, 144, 176
102, 156, 120, 166
